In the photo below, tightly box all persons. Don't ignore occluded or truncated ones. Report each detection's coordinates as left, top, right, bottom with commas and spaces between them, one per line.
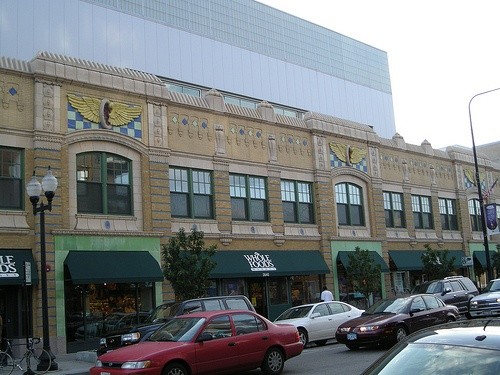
321, 286, 334, 302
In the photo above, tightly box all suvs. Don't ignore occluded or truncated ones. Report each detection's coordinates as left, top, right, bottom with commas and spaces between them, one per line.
412, 275, 479, 316
469, 279, 500, 316
97, 295, 260, 357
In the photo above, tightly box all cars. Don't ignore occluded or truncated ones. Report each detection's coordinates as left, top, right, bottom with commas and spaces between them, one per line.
274, 301, 366, 348
90, 310, 304, 375
335, 294, 460, 350
358, 316, 500, 375
75, 312, 151, 342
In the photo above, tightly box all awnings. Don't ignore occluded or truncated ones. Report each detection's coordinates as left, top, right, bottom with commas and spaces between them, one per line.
65, 250, 164, 285
0, 247, 39, 287
474, 251, 500, 268
337, 250, 387, 273
389, 249, 468, 270
170, 249, 330, 279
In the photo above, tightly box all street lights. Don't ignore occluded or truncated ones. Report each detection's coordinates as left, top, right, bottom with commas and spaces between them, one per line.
27, 165, 60, 371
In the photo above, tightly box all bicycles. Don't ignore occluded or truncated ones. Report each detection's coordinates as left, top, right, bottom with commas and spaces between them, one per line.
0, 337, 52, 375
